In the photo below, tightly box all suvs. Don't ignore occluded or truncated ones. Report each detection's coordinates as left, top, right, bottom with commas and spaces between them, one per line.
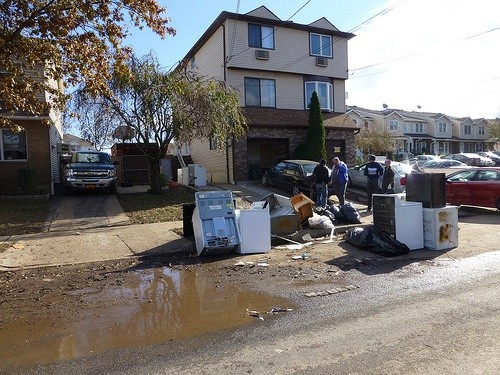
61, 150, 121, 194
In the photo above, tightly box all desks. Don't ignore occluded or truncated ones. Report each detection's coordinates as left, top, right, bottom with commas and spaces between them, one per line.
292, 192, 315, 229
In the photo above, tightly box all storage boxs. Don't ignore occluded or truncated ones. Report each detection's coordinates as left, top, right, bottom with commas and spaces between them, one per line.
405, 172, 458, 251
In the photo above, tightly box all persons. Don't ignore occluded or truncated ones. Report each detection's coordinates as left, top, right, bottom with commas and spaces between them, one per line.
382, 159, 396, 194
364, 155, 383, 213
330, 157, 348, 206
313, 159, 330, 210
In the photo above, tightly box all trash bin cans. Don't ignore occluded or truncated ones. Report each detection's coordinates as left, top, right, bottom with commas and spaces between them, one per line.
181, 203, 196, 243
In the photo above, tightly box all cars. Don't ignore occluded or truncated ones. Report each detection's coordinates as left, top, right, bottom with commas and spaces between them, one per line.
445, 167, 500, 211
260, 160, 338, 202
400, 150, 500, 169
347, 161, 415, 195
